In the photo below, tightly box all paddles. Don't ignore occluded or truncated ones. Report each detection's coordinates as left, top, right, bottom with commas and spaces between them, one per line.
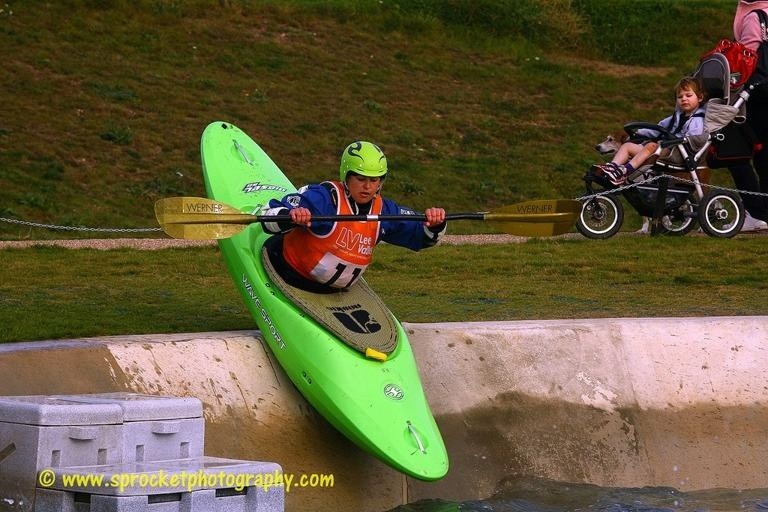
154, 196, 583, 240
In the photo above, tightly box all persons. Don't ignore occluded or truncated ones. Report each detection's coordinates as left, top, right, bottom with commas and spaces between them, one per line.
722, 1, 767, 233
261, 139, 447, 291
592, 75, 707, 187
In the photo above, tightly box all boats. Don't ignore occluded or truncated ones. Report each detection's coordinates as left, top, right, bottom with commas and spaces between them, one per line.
199, 120, 450, 481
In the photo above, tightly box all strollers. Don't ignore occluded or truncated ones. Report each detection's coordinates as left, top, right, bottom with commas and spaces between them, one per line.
574, 52, 766, 240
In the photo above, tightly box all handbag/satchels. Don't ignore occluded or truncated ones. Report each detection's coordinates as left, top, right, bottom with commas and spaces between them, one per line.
701, 39, 758, 88
745, 7, 768, 111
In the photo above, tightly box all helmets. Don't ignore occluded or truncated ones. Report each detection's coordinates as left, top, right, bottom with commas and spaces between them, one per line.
340, 141, 387, 182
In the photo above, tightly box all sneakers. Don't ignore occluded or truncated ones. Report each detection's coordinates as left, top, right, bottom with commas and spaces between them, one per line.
722, 211, 768, 231
593, 162, 627, 185
